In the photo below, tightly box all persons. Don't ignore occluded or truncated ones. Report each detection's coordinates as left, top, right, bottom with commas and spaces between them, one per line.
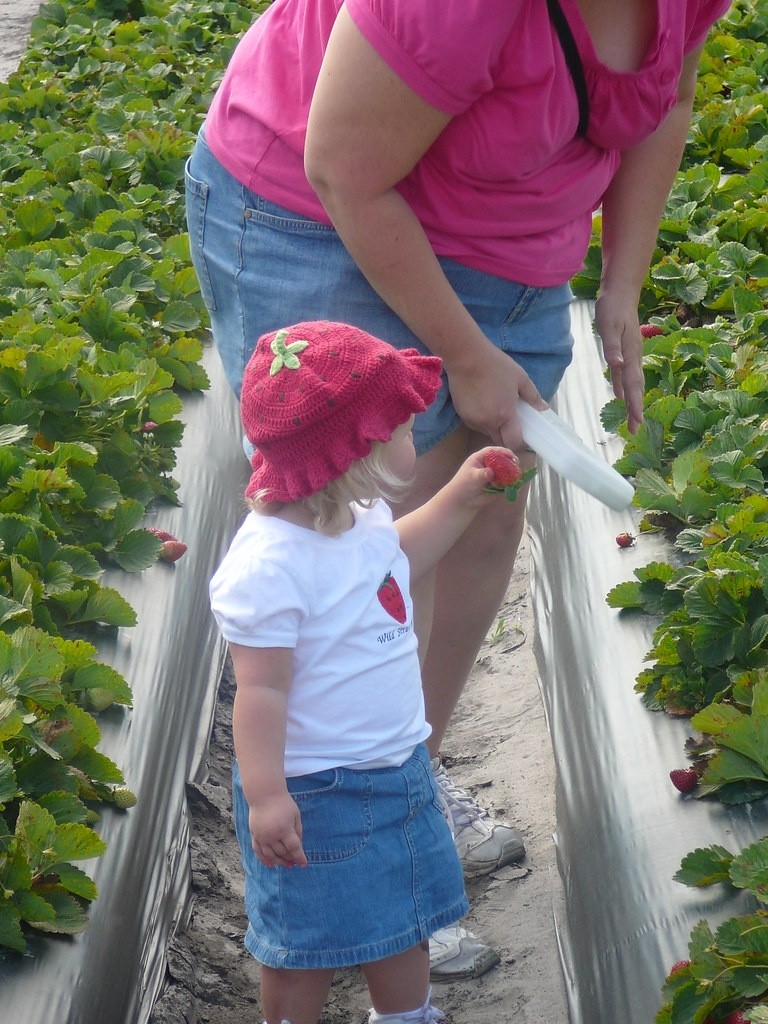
183, 0, 735, 983
209, 322, 521, 1024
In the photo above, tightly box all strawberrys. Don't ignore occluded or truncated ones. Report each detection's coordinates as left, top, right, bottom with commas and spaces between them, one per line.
615, 323, 753, 1024
485, 448, 523, 491
33, 415, 190, 884
379, 575, 409, 623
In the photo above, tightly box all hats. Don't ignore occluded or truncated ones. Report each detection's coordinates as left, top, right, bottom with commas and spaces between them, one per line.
239, 321, 443, 505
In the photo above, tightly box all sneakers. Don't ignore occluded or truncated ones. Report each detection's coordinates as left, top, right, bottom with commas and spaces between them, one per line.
428, 927, 501, 983
428, 757, 525, 879
